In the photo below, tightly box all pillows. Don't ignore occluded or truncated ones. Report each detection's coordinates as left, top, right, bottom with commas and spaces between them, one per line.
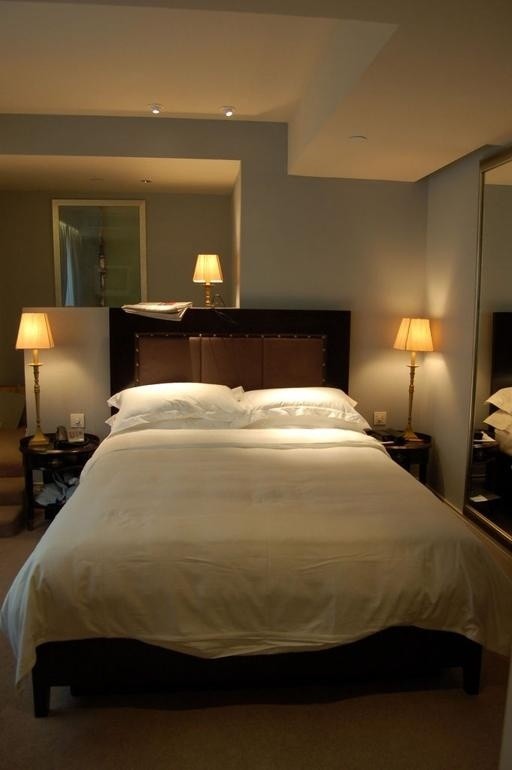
482, 409, 511, 434
232, 386, 373, 435
103, 381, 254, 441
484, 387, 511, 415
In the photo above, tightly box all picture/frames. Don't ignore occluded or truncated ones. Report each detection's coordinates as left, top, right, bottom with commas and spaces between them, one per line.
50, 198, 148, 308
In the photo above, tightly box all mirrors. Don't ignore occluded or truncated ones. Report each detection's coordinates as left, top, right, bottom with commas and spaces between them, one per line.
461, 149, 511, 548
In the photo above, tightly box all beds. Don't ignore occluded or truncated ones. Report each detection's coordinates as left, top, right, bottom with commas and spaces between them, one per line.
1, 305, 511, 721
488, 310, 512, 493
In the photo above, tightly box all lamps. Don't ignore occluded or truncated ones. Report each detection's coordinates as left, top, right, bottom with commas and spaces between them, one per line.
191, 253, 223, 307
15, 312, 54, 446
392, 317, 434, 442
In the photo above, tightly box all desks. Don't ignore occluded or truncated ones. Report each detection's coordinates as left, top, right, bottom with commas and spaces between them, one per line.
368, 430, 433, 488
18, 431, 101, 531
472, 439, 499, 496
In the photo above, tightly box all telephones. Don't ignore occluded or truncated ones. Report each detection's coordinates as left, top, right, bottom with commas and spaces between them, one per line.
56, 426, 85, 444
